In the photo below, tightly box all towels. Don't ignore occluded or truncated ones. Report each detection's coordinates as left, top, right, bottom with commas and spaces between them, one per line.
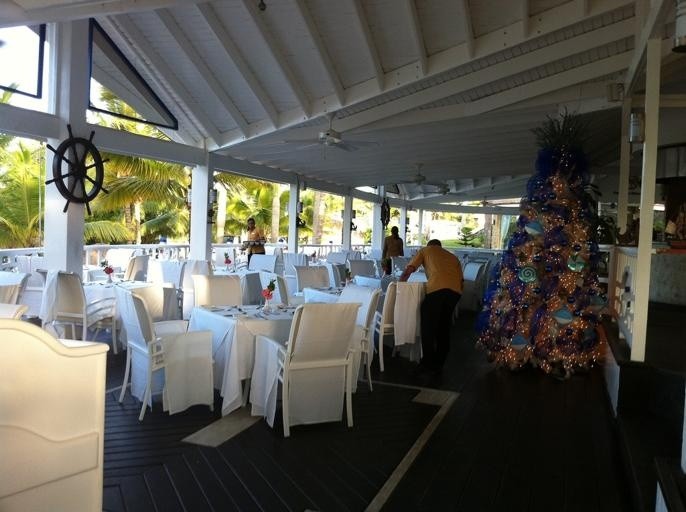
235, 312, 275, 337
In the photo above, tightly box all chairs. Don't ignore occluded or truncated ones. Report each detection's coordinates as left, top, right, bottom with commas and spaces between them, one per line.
92, 255, 153, 340
112, 285, 216, 423
0, 302, 28, 318
340, 284, 381, 393
150, 260, 187, 320
104, 248, 135, 272
35, 268, 119, 354
376, 282, 425, 372
15, 255, 43, 287
293, 264, 330, 295
215, 248, 235, 268
332, 263, 350, 287
326, 250, 362, 262
191, 273, 241, 307
259, 269, 295, 310
249, 253, 278, 273
284, 253, 309, 274
457, 251, 496, 306
181, 257, 212, 320
251, 303, 361, 438
347, 258, 377, 278
355, 276, 381, 289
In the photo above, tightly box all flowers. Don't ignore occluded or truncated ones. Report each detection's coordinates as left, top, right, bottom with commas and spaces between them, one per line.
225, 252, 231, 264
101, 259, 113, 274
346, 268, 352, 282
262, 279, 277, 300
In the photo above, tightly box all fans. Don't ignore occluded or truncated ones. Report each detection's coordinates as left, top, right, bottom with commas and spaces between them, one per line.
421, 178, 466, 196
395, 164, 449, 187
288, 112, 381, 158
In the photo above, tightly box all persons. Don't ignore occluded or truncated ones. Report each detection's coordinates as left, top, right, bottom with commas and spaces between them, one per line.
400, 238, 463, 373
381, 227, 405, 275
246, 216, 268, 267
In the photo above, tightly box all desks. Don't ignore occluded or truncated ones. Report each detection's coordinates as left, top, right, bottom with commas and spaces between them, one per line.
188, 305, 296, 418
53, 280, 156, 339
213, 267, 270, 306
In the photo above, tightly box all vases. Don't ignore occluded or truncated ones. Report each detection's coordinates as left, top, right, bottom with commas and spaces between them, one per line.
226, 265, 229, 272
107, 275, 112, 283
262, 300, 272, 315
